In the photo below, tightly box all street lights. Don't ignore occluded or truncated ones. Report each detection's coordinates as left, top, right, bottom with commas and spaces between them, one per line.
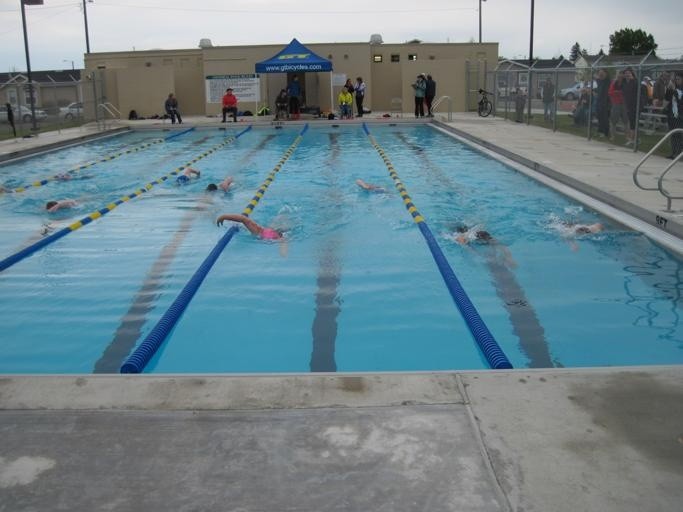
20, 0, 45, 131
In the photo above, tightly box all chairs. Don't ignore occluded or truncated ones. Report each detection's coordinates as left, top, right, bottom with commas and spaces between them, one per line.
388, 97, 403, 117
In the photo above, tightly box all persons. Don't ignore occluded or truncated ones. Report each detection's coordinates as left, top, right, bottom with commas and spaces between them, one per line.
166, 91, 184, 124
556, 219, 600, 254
410, 71, 437, 119
216, 213, 293, 257
541, 78, 557, 121
0, 186, 25, 194
337, 76, 365, 121
514, 87, 527, 123
272, 76, 304, 121
31, 196, 80, 250
220, 88, 238, 122
176, 166, 238, 198
356, 177, 388, 202
569, 67, 683, 160
452, 220, 516, 268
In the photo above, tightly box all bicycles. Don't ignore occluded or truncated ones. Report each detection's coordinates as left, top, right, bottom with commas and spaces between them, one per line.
478, 88, 494, 118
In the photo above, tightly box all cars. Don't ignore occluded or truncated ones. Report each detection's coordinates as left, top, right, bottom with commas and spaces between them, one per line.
557, 80, 598, 101
58, 102, 83, 121
0, 104, 48, 125
496, 79, 530, 101
